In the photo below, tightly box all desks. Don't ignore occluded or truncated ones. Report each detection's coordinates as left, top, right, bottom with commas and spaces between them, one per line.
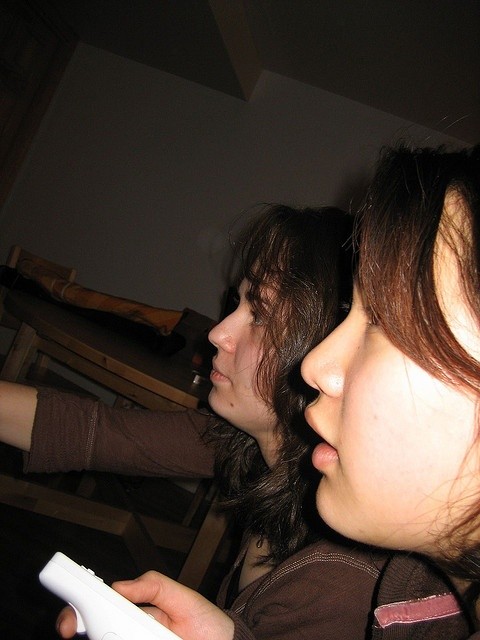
0, 287, 259, 594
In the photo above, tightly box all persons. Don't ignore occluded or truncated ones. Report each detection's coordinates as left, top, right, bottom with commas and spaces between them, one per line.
55, 144, 480, 639
0, 206, 359, 594
